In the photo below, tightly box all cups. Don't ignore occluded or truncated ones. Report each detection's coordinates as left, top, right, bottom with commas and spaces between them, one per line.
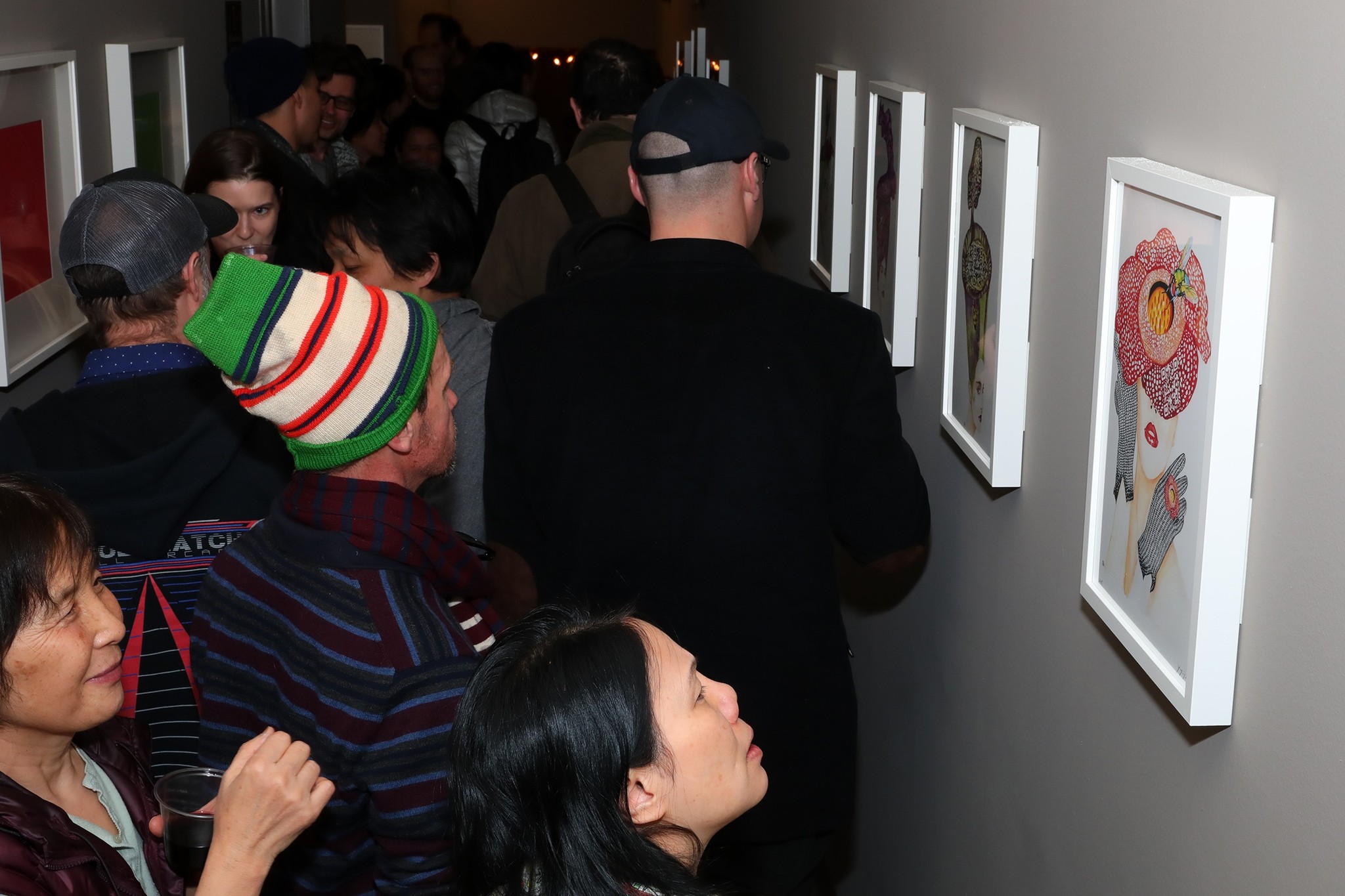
225, 243, 273, 266
151, 765, 231, 888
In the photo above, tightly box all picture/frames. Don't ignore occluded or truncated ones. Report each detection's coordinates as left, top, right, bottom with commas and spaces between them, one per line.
672, 27, 728, 87
345, 24, 385, 65
940, 107, 1041, 488
810, 62, 856, 293
105, 35, 190, 190
1081, 154, 1275, 729
862, 80, 926, 368
259, 1, 310, 48
0, 50, 84, 388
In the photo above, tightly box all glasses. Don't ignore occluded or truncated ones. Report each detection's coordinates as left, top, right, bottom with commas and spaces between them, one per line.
318, 90, 358, 113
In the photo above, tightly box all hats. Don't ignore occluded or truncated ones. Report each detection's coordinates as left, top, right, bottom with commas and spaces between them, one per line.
629, 74, 791, 175
182, 250, 438, 472
58, 166, 240, 299
224, 36, 312, 120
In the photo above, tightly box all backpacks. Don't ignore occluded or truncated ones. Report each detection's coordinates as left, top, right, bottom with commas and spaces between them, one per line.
544, 159, 652, 293
460, 112, 554, 242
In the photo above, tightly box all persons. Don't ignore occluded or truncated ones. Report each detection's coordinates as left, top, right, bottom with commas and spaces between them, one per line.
1, 474, 337, 896
477, 75, 935, 894
182, 252, 543, 896
447, 602, 770, 896
2, 158, 300, 791
1, 11, 671, 547
